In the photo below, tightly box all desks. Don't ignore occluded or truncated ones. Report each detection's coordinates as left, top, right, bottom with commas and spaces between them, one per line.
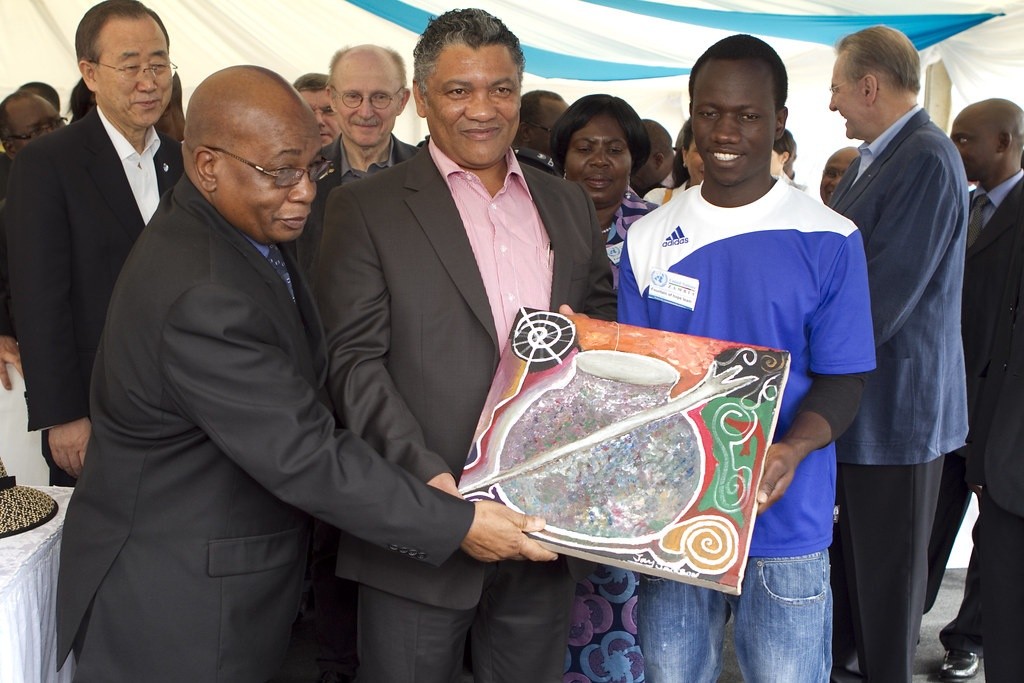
0, 485, 75, 683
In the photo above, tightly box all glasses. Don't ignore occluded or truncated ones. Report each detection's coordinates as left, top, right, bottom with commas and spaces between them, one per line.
5, 116, 68, 139
822, 170, 845, 178
330, 84, 404, 109
89, 59, 178, 81
830, 76, 879, 96
205, 146, 333, 187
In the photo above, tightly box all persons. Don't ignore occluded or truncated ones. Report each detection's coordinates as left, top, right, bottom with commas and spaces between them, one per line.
320, 7, 618, 682
55, 65, 558, 683
0, 1, 1024, 683
617, 34, 876, 682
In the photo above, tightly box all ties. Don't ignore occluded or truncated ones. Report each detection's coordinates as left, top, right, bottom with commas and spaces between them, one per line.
966, 193, 991, 249
267, 245, 297, 301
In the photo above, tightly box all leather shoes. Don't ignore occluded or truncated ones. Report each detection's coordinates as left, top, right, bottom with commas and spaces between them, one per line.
939, 651, 981, 681
